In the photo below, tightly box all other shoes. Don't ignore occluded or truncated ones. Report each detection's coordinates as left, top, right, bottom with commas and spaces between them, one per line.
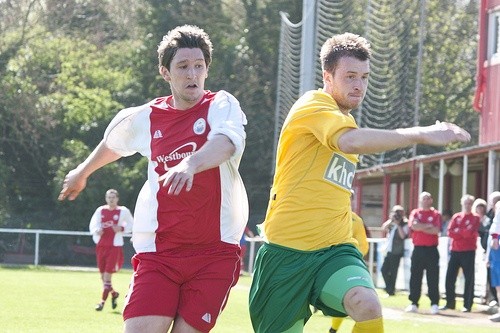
489, 313, 500, 322
477, 299, 499, 315
430, 304, 440, 314
384, 291, 395, 297
96, 303, 104, 311
404, 304, 420, 314
462, 307, 471, 312
438, 305, 455, 310
111, 292, 119, 309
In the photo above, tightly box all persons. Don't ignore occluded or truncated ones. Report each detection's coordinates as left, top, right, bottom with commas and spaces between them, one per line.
61, 24, 249, 333
89, 189, 134, 311
327, 188, 500, 333
249, 32, 471, 333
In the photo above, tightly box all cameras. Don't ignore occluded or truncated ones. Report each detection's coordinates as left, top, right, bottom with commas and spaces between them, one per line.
394, 213, 401, 220
